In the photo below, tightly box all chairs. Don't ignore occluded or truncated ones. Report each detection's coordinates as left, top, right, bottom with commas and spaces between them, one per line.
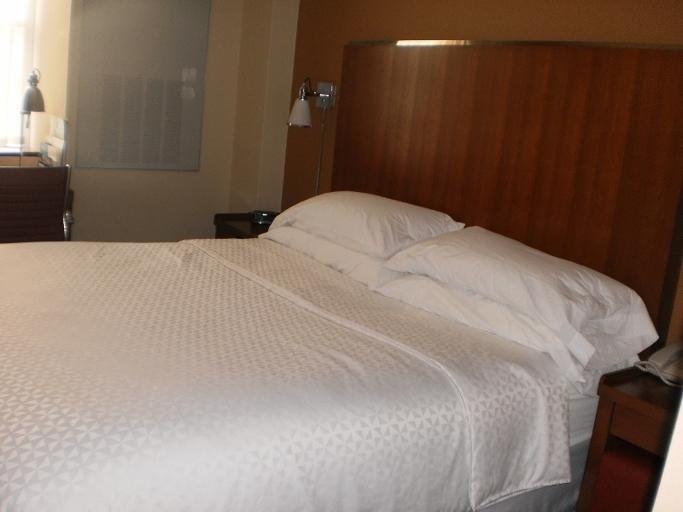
0, 162, 74, 243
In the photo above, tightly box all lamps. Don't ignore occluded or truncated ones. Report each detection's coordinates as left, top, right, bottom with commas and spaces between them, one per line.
287, 76, 337, 195
17, 69, 45, 167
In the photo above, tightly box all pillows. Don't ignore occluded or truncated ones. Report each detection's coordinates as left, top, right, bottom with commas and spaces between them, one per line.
264, 189, 463, 283
386, 224, 661, 368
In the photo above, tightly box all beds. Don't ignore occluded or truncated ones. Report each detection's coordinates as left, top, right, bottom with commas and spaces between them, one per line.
0, 40, 682, 512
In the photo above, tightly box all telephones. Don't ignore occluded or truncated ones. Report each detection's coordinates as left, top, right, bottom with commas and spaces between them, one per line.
644, 341, 683, 382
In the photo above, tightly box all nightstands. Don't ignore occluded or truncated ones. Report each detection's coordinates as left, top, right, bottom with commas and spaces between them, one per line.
212, 213, 280, 240
576, 364, 681, 512
